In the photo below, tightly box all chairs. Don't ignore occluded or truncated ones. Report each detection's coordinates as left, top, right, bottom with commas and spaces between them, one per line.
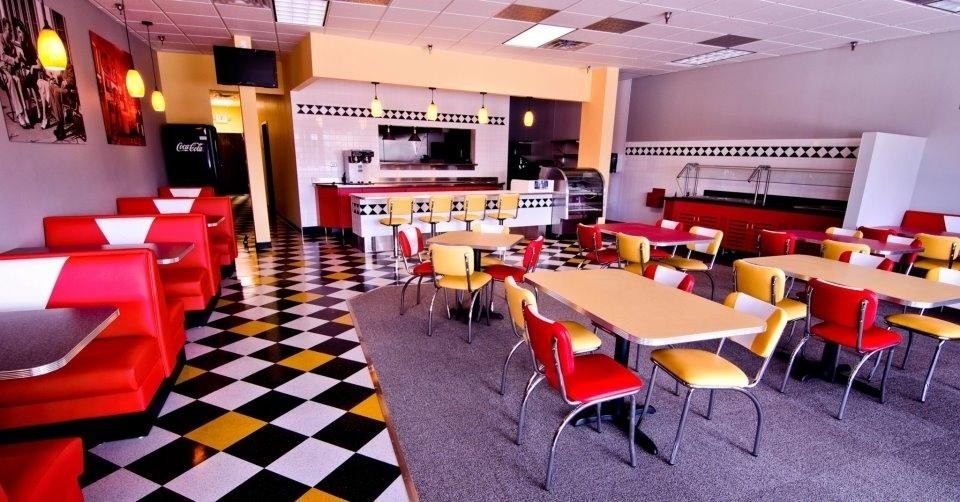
634, 292, 787, 466
857, 226, 895, 246
516, 301, 644, 489
660, 227, 723, 301
648, 218, 683, 262
379, 197, 412, 260
757, 229, 794, 257
472, 224, 508, 266
905, 233, 960, 279
826, 228, 861, 239
839, 252, 894, 272
733, 260, 808, 362
820, 240, 870, 265
477, 237, 543, 321
397, 229, 461, 315
869, 267, 960, 402
616, 233, 674, 279
577, 224, 623, 269
427, 245, 492, 342
590, 263, 694, 371
455, 194, 485, 233
781, 278, 902, 420
487, 193, 520, 234
419, 196, 453, 258
499, 276, 601, 406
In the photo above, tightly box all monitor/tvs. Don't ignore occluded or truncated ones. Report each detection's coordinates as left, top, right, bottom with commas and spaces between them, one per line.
213, 45, 278, 88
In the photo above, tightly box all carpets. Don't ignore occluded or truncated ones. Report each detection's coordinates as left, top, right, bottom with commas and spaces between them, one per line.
347, 258, 956, 501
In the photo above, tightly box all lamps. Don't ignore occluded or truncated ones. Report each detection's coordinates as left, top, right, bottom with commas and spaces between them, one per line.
407, 128, 420, 141
427, 88, 437, 120
141, 21, 165, 112
370, 82, 383, 116
36, 1, 68, 71
523, 97, 534, 126
122, 0, 145, 97
477, 92, 489, 123
382, 125, 396, 140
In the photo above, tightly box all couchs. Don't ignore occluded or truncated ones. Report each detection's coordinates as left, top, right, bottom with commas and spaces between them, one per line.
0, 250, 186, 384
43, 216, 215, 320
157, 187, 214, 199
116, 197, 236, 276
1, 438, 86, 502
1, 255, 168, 444
44, 214, 221, 297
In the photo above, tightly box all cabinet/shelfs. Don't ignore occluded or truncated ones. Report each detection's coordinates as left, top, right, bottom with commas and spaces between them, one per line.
549, 168, 604, 237
